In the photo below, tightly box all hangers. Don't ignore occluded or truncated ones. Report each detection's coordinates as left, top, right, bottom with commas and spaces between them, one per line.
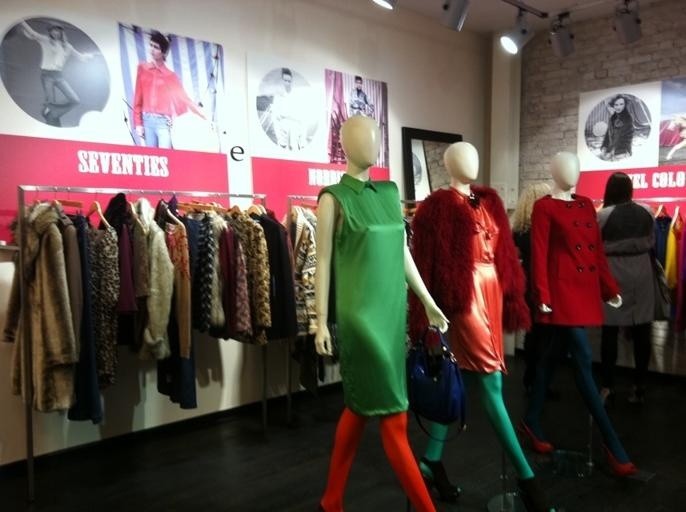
286, 193, 311, 222
27, 184, 269, 233
651, 198, 683, 230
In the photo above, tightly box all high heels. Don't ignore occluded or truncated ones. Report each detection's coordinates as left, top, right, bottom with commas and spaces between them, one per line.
419, 456, 462, 500
517, 477, 557, 512
601, 443, 640, 475
515, 418, 555, 453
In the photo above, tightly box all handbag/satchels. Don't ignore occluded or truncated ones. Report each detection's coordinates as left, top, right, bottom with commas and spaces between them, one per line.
406, 324, 467, 425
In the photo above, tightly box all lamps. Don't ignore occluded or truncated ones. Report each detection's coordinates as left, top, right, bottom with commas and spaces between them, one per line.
497, 2, 646, 61
439, 2, 471, 33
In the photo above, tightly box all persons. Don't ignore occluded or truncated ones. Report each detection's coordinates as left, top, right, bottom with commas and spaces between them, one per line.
599, 95, 634, 161
349, 76, 374, 116
272, 68, 302, 150
132, 33, 206, 149
20, 21, 93, 128
595, 171, 659, 409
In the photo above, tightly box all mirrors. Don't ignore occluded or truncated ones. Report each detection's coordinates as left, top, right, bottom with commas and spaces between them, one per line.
402, 127, 462, 217
